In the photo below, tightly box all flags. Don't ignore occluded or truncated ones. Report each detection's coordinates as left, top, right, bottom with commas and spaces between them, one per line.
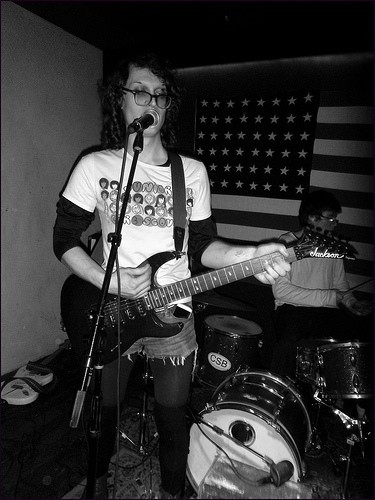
195, 88, 375, 297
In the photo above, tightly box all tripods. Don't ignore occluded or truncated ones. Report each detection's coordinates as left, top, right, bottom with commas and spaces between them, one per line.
115, 361, 158, 456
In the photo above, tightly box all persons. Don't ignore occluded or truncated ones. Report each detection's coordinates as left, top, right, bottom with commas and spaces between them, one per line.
275, 191, 375, 341
52, 56, 293, 500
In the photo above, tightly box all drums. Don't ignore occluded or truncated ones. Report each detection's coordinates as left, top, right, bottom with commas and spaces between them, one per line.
193, 295, 245, 340
199, 313, 263, 388
186, 371, 313, 500
314, 343, 375, 400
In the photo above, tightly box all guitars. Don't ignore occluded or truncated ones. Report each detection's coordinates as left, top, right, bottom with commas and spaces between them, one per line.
0, 337, 72, 405
60, 222, 357, 368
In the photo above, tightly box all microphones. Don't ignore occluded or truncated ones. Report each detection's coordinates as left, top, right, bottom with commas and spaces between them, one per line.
257, 460, 294, 487
127, 109, 160, 133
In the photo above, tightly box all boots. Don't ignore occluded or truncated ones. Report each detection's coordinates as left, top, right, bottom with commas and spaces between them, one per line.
86, 470, 108, 500
159, 484, 185, 500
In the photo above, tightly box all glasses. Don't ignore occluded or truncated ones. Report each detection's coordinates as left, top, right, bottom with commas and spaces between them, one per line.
321, 215, 339, 225
122, 86, 173, 109
